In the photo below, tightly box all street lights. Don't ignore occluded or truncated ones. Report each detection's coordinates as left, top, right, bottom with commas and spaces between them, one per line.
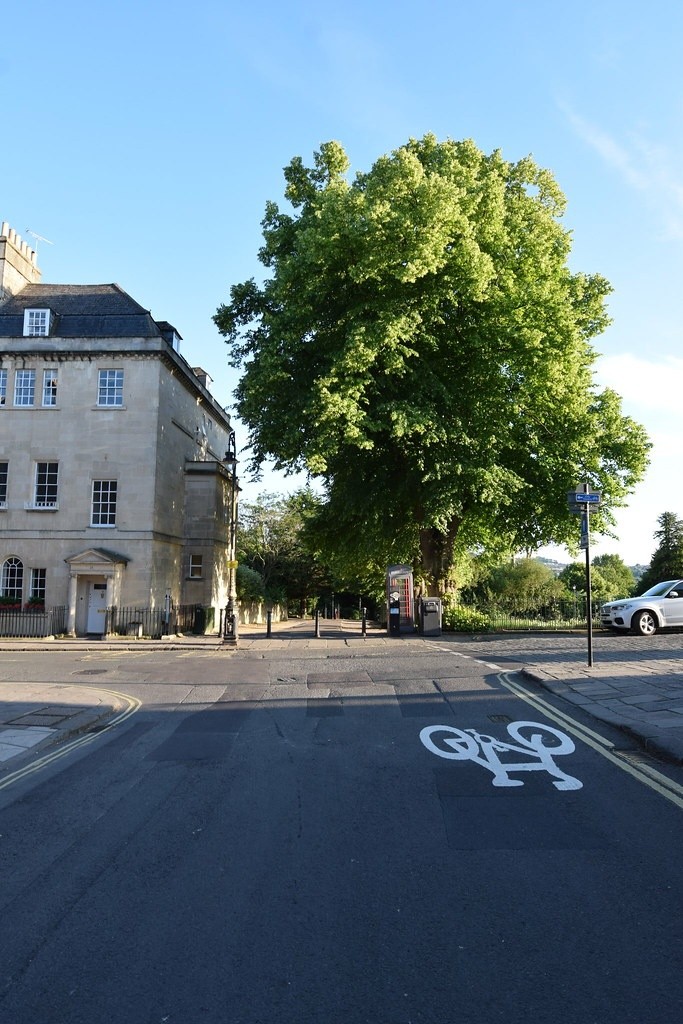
221, 430, 241, 644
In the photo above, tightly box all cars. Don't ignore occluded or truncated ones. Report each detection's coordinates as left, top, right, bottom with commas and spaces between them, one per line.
598, 578, 683, 636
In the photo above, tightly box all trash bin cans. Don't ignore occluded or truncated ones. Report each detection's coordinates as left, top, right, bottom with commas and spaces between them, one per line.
417, 596, 444, 638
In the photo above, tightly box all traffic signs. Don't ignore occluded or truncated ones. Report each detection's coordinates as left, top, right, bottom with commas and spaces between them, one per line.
576, 493, 600, 502
